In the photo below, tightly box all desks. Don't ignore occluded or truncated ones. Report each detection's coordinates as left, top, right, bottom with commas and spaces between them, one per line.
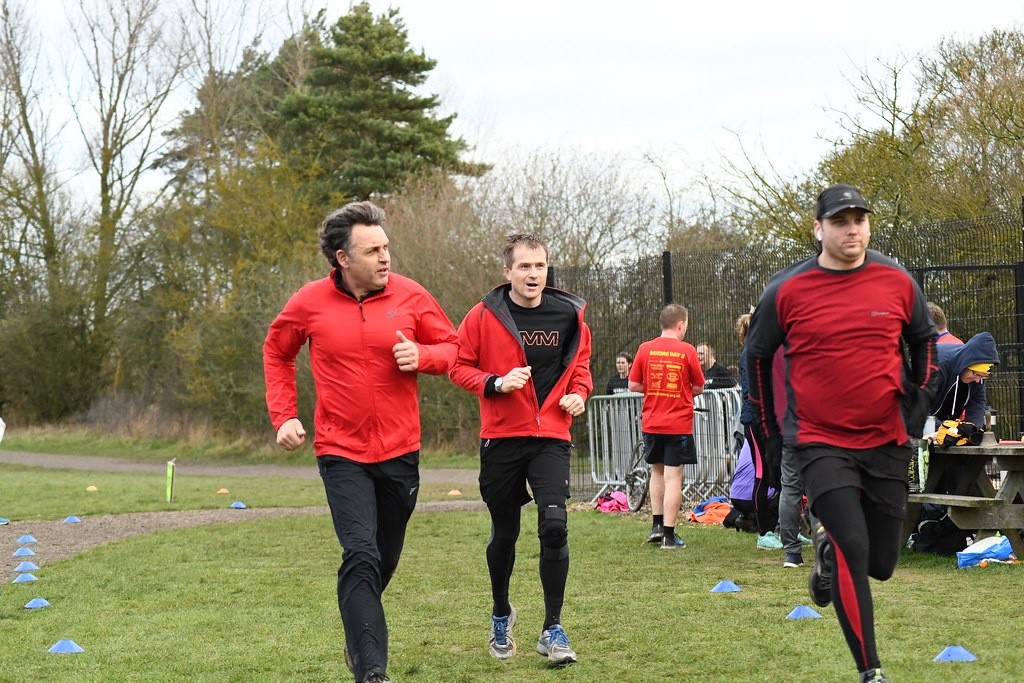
929, 441, 1024, 560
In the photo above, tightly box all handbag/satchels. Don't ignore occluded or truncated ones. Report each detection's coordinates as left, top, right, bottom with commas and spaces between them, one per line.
935, 419, 983, 445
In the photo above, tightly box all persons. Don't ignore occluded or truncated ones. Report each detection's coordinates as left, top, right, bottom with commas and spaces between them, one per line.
697, 342, 738, 463
729, 301, 1000, 549
628, 304, 705, 549
606, 351, 643, 436
266, 201, 459, 682
745, 184, 939, 683
448, 235, 592, 666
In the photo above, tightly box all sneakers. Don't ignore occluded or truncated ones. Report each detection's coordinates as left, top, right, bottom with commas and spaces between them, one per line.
757, 532, 784, 550
782, 553, 803, 568
488, 601, 517, 660
647, 524, 663, 542
660, 533, 687, 550
860, 668, 887, 683
536, 625, 576, 663
808, 534, 833, 607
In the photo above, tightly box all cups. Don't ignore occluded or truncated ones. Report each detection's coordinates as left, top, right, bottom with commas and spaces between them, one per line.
922, 416, 935, 438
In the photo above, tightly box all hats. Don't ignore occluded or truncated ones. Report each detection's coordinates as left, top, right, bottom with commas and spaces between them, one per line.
967, 363, 994, 374
816, 185, 873, 218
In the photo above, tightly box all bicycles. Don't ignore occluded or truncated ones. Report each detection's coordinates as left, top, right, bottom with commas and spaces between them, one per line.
626, 407, 709, 514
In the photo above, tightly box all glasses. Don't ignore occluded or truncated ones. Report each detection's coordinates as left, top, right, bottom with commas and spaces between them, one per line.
972, 370, 988, 380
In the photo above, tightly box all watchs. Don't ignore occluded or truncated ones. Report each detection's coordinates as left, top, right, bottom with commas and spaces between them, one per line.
495, 376, 503, 393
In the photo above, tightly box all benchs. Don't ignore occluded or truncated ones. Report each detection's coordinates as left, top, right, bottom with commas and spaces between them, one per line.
906, 493, 1005, 508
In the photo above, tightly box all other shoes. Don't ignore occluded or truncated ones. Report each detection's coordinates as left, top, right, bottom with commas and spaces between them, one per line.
361, 665, 390, 683
343, 643, 355, 669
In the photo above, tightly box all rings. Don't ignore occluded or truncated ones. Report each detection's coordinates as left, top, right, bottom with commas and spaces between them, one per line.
573, 410, 575, 414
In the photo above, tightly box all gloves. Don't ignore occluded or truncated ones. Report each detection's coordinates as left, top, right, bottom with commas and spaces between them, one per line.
902, 380, 934, 440
762, 436, 782, 470
